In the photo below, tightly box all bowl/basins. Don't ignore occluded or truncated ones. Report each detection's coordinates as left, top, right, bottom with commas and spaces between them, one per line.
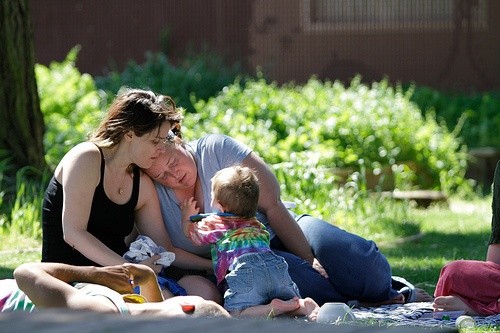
316, 303, 356, 322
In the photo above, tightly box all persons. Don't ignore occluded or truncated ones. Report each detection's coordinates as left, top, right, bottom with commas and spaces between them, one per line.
432, 159, 500, 314
13, 89, 222, 304
145, 132, 405, 307
0, 262, 233, 323
180, 165, 319, 322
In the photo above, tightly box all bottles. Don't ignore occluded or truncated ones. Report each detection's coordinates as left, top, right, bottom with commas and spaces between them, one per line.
455, 315, 475, 329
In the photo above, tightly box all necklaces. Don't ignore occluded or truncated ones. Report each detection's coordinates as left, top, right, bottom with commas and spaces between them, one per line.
117, 172, 126, 195
